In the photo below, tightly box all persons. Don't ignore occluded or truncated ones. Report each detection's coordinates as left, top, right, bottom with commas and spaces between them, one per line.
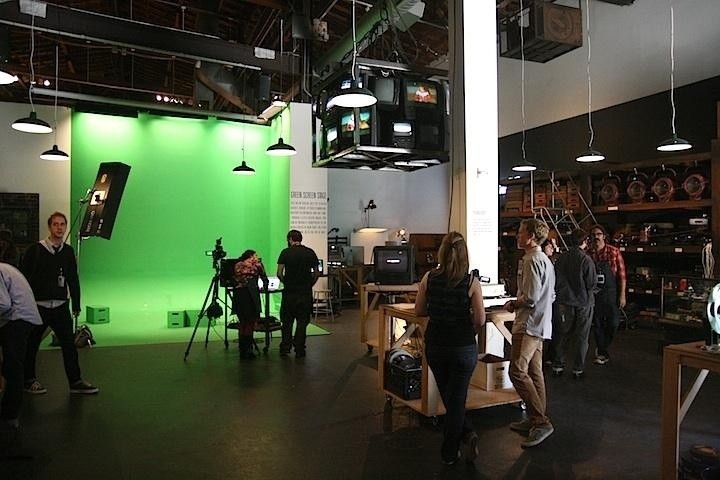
503, 218, 557, 449
276, 230, 321, 358
232, 250, 268, 349
586, 224, 628, 366
552, 227, 599, 376
0, 263, 43, 430
22, 211, 99, 395
414, 231, 486, 467
542, 239, 554, 366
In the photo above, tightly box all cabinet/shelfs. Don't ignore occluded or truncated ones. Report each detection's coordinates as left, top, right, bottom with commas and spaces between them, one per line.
229, 287, 284, 345
357, 283, 503, 352
659, 273, 716, 330
329, 263, 372, 301
588, 172, 714, 334
383, 301, 532, 424
494, 178, 579, 317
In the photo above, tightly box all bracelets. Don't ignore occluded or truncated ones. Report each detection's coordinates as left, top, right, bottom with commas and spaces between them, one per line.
510, 300, 518, 310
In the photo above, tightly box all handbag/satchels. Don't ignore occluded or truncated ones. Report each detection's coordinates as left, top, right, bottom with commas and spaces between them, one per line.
74, 324, 92, 349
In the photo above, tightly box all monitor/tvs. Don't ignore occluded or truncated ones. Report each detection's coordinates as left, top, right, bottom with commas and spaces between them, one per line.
319, 129, 327, 159
402, 79, 447, 120
380, 113, 447, 152
374, 245, 416, 287
372, 77, 402, 112
326, 124, 343, 156
339, 107, 373, 146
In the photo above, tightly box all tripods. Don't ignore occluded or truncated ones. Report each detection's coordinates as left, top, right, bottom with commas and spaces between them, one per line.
183, 260, 261, 361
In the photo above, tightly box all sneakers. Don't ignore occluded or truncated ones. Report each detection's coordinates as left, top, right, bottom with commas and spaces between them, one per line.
21, 380, 47, 394
69, 380, 99, 394
442, 431, 480, 462
516, 416, 555, 445
548, 354, 612, 374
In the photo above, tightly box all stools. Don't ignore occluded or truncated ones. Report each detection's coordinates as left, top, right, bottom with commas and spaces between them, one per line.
312, 290, 335, 323
86, 305, 109, 324
166, 309, 185, 329
186, 308, 216, 327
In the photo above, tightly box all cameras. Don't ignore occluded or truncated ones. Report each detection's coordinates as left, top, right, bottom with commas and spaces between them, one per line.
206, 237, 228, 258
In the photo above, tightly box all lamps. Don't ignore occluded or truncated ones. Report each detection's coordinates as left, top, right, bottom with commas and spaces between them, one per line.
0, 0, 17, 88
507, 4, 538, 172
38, 44, 69, 161
13, 5, 55, 136
234, 68, 257, 177
355, 206, 390, 234
335, 1, 377, 110
269, 17, 297, 158
656, 7, 695, 153
576, 2, 606, 164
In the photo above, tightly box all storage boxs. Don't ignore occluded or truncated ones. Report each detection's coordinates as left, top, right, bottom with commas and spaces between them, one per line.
471, 352, 515, 392
505, 185, 579, 212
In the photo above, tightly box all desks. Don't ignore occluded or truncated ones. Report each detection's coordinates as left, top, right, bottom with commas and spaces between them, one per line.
659, 343, 719, 479
